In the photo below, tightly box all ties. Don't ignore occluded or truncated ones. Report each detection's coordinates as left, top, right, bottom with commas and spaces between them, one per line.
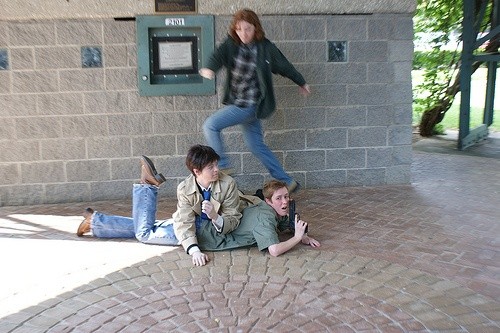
201, 190, 211, 219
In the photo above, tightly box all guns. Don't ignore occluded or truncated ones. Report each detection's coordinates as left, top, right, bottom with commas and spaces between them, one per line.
288, 200, 308, 234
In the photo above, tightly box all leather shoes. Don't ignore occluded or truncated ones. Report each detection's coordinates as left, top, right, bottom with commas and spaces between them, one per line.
140, 154, 167, 185
77, 207, 94, 236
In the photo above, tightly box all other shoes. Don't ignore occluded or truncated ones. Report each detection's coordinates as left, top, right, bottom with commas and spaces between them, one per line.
287, 179, 300, 194
221, 168, 236, 177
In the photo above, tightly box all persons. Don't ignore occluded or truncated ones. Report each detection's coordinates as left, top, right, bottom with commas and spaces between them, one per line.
172, 144, 264, 267
78, 155, 321, 257
197, 8, 311, 195
